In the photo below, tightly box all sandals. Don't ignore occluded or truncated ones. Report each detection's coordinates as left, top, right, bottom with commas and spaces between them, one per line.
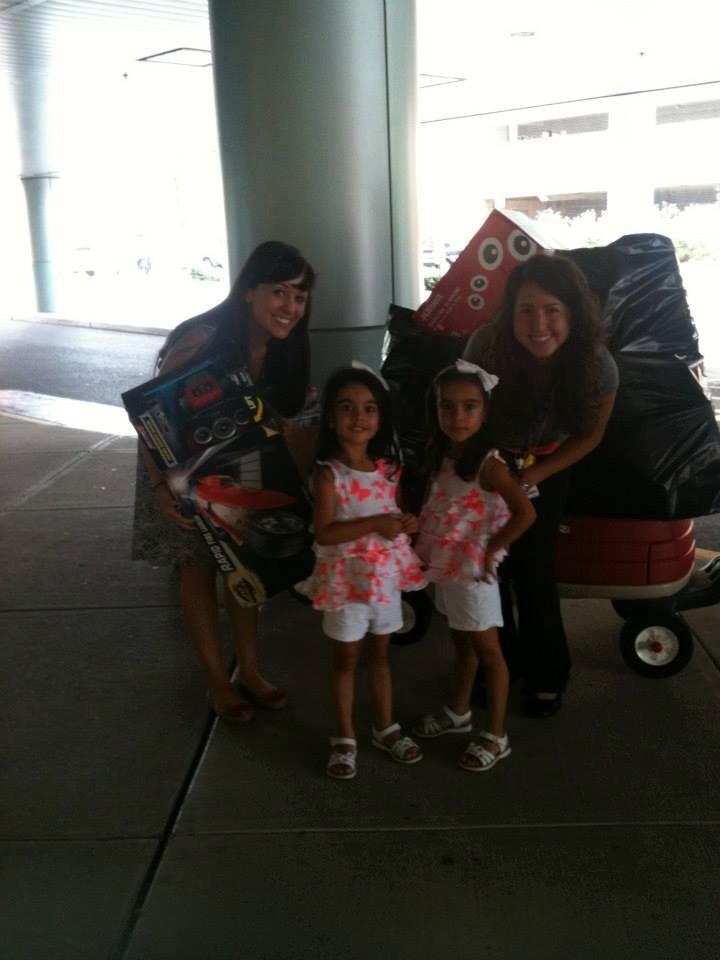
459, 729, 512, 771
413, 705, 472, 737
327, 739, 358, 780
371, 723, 423, 764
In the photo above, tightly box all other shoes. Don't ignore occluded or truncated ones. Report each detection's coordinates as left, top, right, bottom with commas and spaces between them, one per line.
529, 694, 562, 717
245, 684, 286, 710
206, 689, 253, 721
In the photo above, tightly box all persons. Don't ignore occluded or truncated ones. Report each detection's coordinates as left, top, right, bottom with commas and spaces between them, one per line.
414, 358, 537, 771
294, 367, 428, 779
461, 253, 619, 718
129, 241, 318, 726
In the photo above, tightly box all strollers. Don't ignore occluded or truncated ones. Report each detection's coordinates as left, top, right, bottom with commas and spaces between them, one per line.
380, 232, 720, 679
